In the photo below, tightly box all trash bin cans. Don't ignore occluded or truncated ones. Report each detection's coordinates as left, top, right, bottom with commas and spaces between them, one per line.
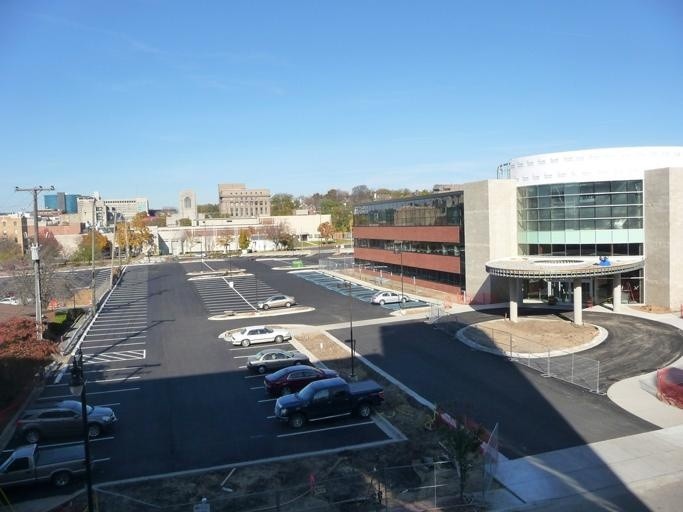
112, 267, 121, 284
291, 261, 303, 267
548, 295, 555, 305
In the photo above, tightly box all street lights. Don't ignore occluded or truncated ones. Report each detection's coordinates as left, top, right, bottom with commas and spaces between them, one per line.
71, 350, 91, 512
336, 283, 353, 376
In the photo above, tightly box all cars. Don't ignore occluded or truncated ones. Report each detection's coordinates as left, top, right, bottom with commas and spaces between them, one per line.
257, 295, 295, 310
264, 364, 336, 396
16, 399, 116, 444
231, 325, 291, 347
245, 348, 309, 373
371, 289, 409, 305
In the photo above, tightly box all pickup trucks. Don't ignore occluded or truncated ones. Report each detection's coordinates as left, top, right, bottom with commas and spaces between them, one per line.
0, 444, 93, 486
274, 378, 383, 429
